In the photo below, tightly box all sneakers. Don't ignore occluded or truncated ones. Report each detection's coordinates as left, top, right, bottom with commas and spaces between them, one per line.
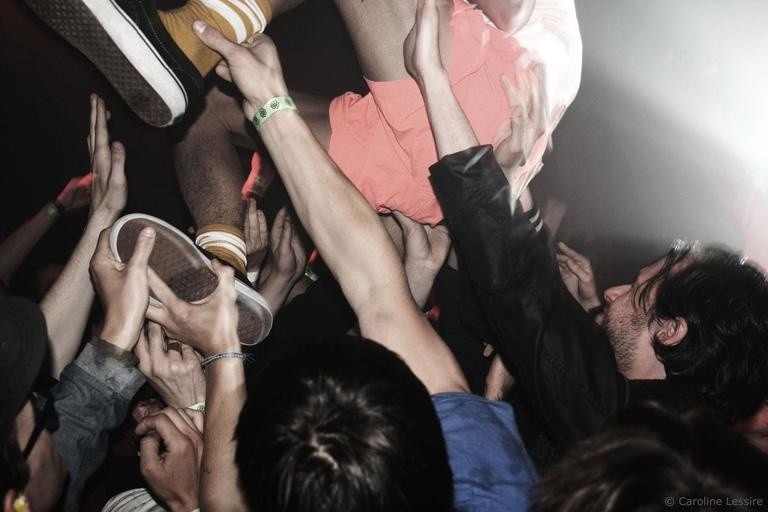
108, 210, 273, 348
0, 0, 206, 129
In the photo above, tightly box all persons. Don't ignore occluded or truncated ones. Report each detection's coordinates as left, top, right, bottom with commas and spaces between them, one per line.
1, 1, 767, 511
